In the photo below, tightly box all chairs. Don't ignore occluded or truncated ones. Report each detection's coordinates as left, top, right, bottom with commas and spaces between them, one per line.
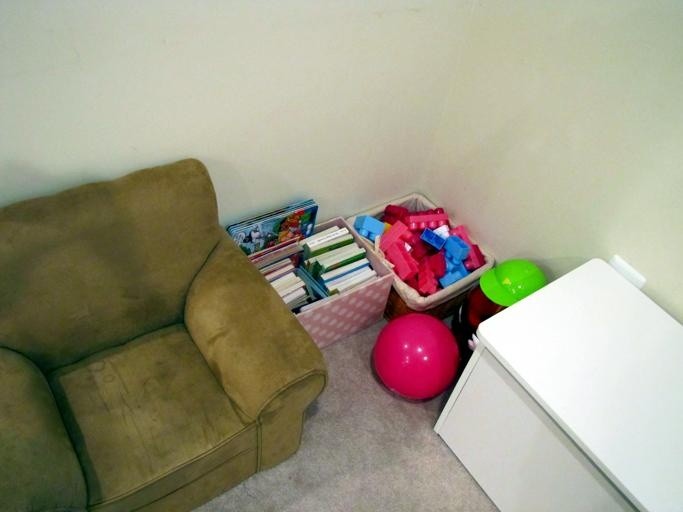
0, 157, 328, 512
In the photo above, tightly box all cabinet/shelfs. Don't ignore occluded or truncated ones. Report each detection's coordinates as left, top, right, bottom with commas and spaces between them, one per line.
434, 256, 683, 512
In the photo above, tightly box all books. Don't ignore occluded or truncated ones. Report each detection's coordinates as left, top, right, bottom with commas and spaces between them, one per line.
246, 225, 380, 316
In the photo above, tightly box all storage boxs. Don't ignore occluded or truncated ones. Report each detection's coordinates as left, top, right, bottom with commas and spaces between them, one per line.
246, 216, 395, 346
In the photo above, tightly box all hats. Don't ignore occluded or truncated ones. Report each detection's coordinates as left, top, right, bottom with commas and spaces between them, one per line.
479, 257, 548, 308
